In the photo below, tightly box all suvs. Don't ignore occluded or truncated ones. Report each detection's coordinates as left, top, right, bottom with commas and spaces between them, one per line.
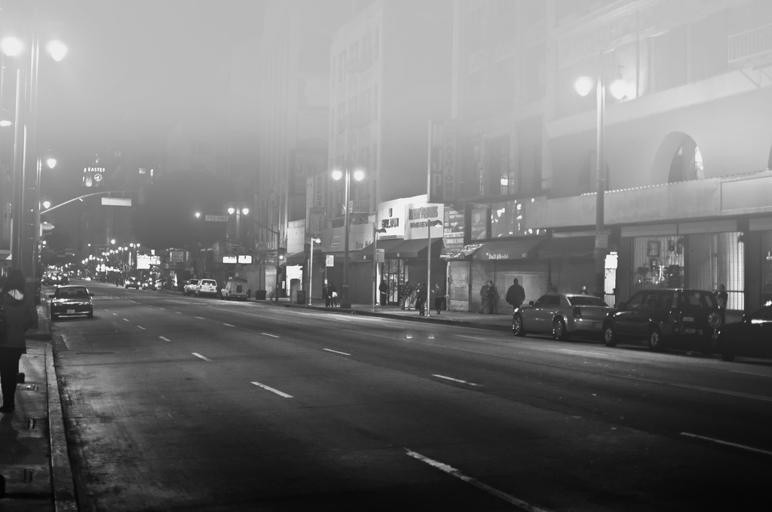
183, 278, 198, 296
194, 278, 218, 298
600, 286, 726, 354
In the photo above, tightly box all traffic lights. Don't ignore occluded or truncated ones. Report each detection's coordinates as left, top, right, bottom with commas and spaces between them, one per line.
278, 247, 285, 261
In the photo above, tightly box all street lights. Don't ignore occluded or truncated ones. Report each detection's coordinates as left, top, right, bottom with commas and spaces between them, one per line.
0, 27, 75, 330
226, 206, 250, 238
425, 217, 445, 316
0, 117, 60, 259
328, 164, 367, 307
79, 238, 147, 269
371, 226, 388, 312
306, 236, 324, 306
571, 60, 634, 298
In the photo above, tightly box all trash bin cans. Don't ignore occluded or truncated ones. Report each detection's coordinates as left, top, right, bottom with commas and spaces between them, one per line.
297, 291, 306, 304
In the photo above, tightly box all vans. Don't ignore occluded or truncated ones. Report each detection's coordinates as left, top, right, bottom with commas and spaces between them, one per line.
219, 274, 250, 300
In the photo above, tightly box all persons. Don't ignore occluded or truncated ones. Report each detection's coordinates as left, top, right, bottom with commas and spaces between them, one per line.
0, 270, 39, 413
328, 282, 338, 308
486, 280, 496, 314
480, 282, 490, 314
379, 280, 442, 316
714, 284, 727, 323
505, 279, 525, 312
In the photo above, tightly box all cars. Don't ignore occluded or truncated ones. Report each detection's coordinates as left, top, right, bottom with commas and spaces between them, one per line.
719, 297, 772, 364
47, 284, 96, 321
40, 268, 70, 286
508, 290, 619, 342
114, 274, 160, 291
